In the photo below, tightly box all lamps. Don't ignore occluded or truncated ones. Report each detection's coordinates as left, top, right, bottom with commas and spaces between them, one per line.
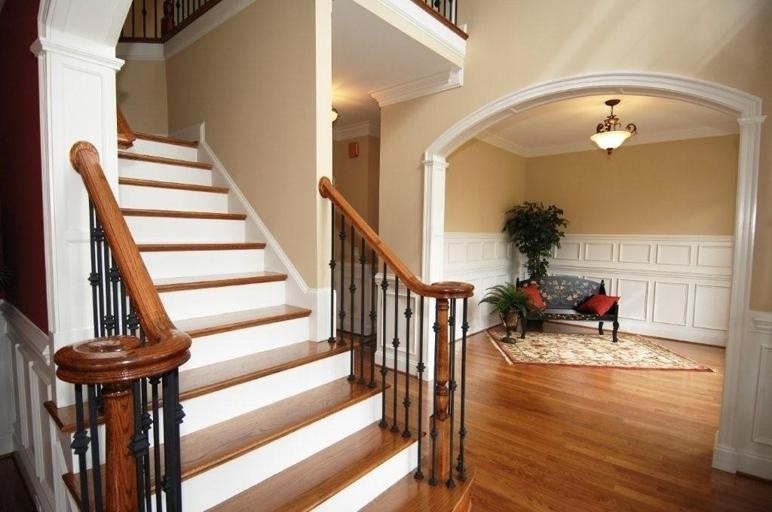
591, 100, 637, 155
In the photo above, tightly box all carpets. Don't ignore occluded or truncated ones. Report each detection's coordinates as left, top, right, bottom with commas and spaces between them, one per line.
483, 329, 714, 374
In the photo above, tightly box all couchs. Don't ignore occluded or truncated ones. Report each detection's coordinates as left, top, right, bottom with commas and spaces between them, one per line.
515, 276, 620, 344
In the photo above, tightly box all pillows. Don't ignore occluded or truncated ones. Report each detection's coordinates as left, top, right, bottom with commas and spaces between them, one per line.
577, 295, 620, 317
520, 283, 547, 313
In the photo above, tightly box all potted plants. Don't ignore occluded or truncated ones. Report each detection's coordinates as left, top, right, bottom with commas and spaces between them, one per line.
503, 197, 567, 333
482, 283, 530, 346
